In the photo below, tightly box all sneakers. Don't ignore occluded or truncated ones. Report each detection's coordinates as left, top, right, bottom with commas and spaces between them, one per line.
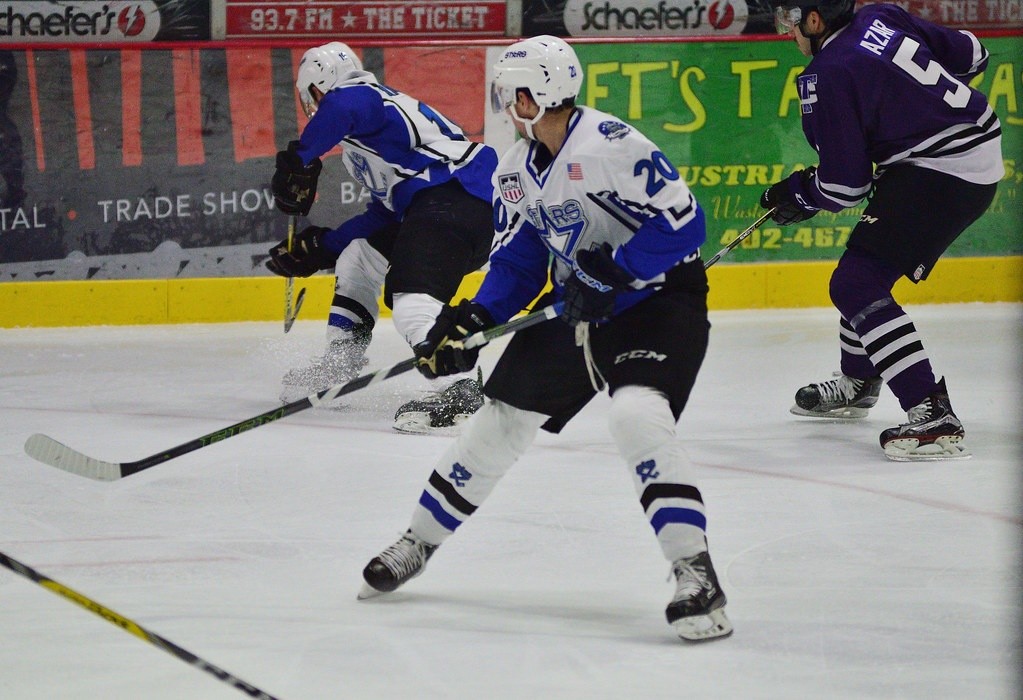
787, 369, 886, 420
879, 374, 974, 461
665, 550, 733, 642
391, 377, 485, 437
355, 525, 442, 602
277, 337, 373, 410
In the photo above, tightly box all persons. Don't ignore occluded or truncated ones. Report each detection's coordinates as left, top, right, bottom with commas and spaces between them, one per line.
757, 0, 1007, 446
271, 42, 498, 422
362, 33, 731, 628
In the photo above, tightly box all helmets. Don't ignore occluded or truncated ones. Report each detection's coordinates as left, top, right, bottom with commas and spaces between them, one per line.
775, 0, 855, 28
492, 35, 584, 110
296, 41, 363, 108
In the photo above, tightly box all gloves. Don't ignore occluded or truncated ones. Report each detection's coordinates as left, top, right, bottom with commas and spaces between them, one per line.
557, 239, 637, 331
270, 140, 324, 219
265, 224, 338, 279
411, 298, 497, 381
759, 165, 824, 228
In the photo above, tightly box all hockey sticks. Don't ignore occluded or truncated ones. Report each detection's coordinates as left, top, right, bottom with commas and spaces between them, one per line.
703, 206, 779, 271
22, 297, 614, 483
284, 212, 306, 333
1, 554, 277, 699
